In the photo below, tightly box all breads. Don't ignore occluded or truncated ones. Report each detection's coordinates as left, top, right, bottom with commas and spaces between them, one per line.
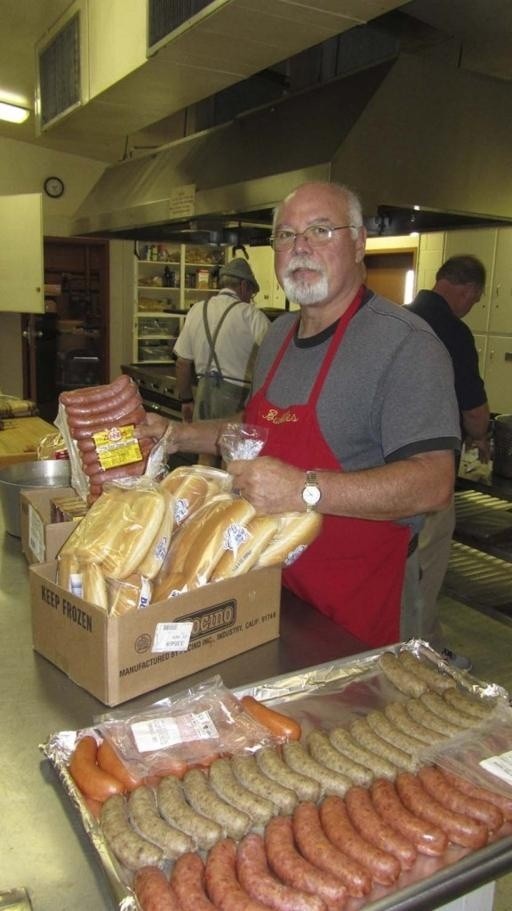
55, 473, 323, 616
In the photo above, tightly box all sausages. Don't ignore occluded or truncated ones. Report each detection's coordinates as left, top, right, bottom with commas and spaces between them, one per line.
69, 650, 512, 911
60, 374, 164, 506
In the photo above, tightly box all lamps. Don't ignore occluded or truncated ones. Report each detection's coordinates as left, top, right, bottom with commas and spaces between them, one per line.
0, 100, 31, 125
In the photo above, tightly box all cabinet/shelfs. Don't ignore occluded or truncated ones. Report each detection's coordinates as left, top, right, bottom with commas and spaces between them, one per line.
472, 332, 511, 415
109, 241, 230, 383
442, 225, 511, 335
225, 243, 300, 320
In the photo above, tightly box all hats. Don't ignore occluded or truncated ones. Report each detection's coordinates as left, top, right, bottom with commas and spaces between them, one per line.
218, 257, 259, 293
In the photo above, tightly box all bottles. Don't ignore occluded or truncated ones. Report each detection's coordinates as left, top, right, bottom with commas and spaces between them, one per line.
168, 271, 176, 286
145, 243, 166, 261
162, 265, 170, 286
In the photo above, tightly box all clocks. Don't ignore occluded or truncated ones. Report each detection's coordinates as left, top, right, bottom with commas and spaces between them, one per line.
44, 176, 64, 198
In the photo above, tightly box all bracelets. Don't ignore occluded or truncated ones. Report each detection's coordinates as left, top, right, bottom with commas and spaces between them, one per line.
178, 395, 194, 403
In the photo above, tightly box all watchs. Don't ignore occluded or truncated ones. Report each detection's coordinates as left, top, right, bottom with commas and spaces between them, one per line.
300, 471, 321, 513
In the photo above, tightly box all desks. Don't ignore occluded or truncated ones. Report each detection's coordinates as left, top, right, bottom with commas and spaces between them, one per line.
1, 531, 511, 911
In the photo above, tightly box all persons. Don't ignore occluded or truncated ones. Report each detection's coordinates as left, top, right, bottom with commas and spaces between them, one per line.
172, 257, 273, 468
400, 254, 492, 670
130, 182, 463, 646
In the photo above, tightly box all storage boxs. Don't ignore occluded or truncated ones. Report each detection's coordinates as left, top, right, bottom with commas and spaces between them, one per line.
18, 485, 88, 565
29, 559, 282, 709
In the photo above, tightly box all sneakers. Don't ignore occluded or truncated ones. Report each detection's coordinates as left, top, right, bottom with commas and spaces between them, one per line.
439, 647, 474, 673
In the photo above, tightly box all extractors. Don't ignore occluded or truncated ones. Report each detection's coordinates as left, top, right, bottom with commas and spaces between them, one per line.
66, 0, 512, 243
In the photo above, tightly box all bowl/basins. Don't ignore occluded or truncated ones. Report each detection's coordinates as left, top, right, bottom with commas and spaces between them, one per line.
142, 344, 173, 360
1, 458, 72, 539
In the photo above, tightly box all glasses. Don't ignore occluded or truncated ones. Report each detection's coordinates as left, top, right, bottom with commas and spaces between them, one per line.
265, 224, 356, 252
